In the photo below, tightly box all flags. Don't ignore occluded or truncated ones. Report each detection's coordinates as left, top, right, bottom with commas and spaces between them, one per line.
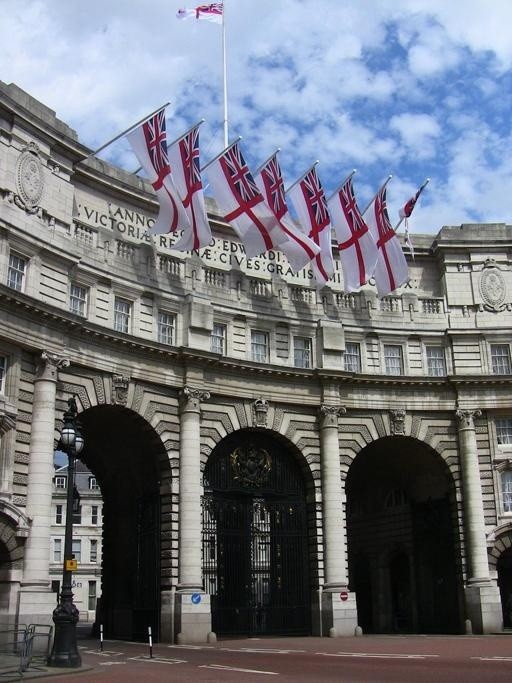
176, 2, 224, 25
398, 188, 419, 259
253, 155, 322, 275
327, 176, 379, 293
289, 168, 334, 291
207, 143, 290, 258
171, 126, 213, 251
363, 187, 409, 297
126, 109, 190, 235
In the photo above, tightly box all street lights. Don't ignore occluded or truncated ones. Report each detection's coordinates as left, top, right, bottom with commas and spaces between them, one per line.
46, 399, 85, 667
